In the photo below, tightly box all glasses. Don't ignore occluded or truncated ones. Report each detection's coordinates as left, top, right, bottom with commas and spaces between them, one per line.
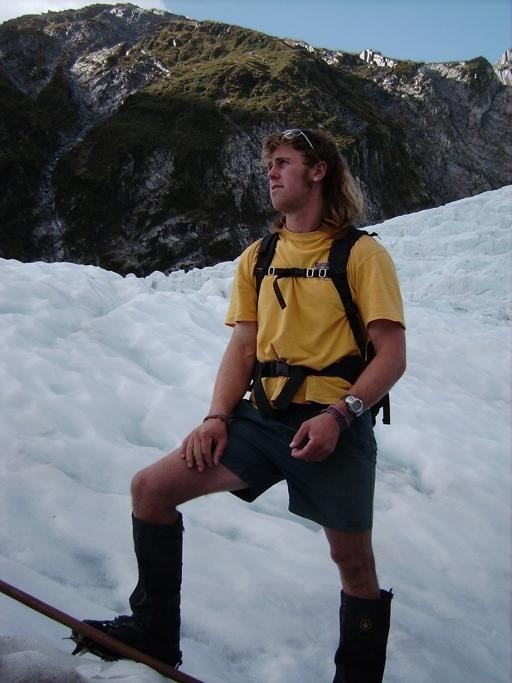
281, 128, 315, 152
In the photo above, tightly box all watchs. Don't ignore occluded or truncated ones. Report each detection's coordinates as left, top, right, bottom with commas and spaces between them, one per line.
341, 394, 366, 419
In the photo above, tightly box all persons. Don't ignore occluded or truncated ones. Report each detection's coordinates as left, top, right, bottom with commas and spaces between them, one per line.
72, 128, 406, 683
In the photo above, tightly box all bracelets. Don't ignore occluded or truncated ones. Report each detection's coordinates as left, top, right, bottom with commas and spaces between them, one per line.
203, 413, 230, 424
320, 405, 350, 432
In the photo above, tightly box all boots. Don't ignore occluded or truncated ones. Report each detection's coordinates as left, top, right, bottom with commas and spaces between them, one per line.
70, 511, 184, 670
332, 587, 393, 683
